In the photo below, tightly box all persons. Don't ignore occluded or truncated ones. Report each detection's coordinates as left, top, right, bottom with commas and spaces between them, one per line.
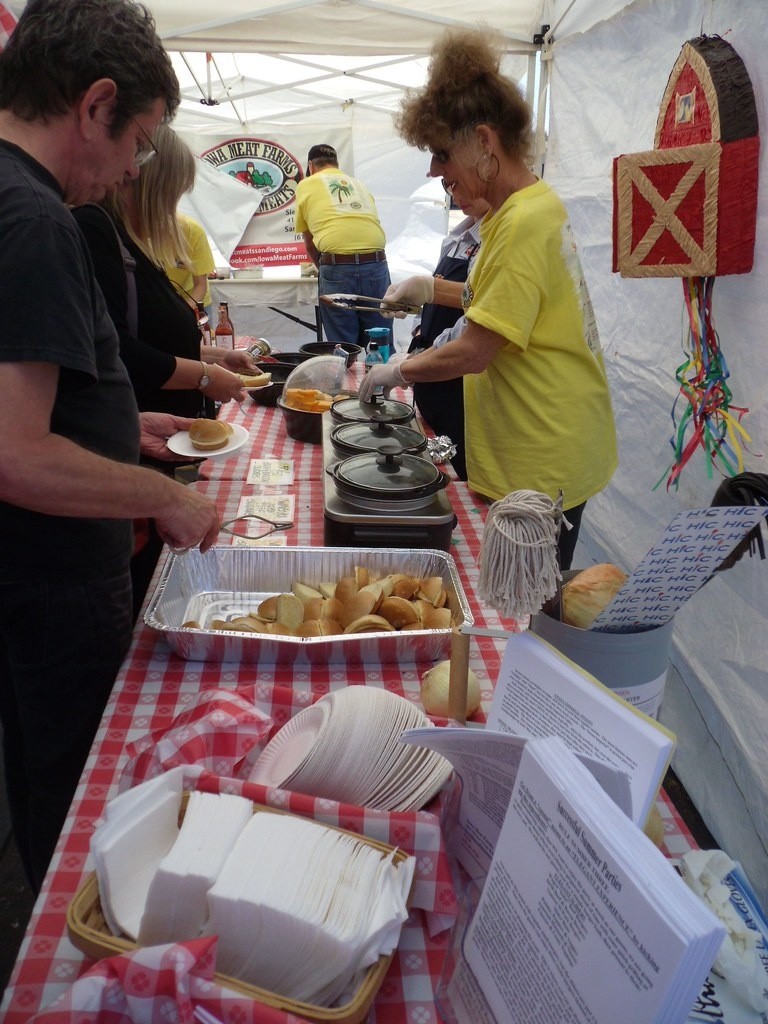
359, 23, 618, 570
144, 205, 215, 327
66, 119, 261, 621
0, 0, 220, 894
294, 144, 395, 356
410, 176, 490, 482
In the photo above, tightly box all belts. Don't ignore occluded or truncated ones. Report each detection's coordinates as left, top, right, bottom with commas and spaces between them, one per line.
319, 250, 387, 264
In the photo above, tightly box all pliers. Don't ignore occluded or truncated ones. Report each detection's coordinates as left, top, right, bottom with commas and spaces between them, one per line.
169, 514, 294, 557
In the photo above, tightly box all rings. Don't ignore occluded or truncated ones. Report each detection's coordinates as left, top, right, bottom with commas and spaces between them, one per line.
174, 547, 186, 552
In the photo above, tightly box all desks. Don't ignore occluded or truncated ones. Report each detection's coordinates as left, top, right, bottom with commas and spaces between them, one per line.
208, 279, 324, 345
0, 361, 704, 1024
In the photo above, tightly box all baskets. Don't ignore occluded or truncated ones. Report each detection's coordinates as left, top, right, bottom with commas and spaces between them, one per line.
65, 784, 416, 1024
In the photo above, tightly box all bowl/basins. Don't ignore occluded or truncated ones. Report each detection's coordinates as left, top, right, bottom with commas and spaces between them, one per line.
300, 341, 361, 370
272, 352, 317, 369
249, 361, 313, 405
275, 394, 327, 444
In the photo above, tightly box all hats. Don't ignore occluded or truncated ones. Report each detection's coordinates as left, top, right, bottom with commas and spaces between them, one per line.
305, 143, 338, 177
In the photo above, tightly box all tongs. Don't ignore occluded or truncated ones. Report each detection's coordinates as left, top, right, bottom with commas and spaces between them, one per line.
217, 514, 295, 539
319, 293, 419, 315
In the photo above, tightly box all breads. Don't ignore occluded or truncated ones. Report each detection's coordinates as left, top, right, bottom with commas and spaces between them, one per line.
188, 419, 234, 451
283, 388, 332, 413
243, 372, 272, 387
559, 563, 626, 628
181, 564, 455, 635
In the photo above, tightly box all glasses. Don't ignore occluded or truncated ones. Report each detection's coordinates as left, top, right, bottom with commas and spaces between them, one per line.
440, 178, 457, 196
128, 114, 158, 167
428, 146, 450, 163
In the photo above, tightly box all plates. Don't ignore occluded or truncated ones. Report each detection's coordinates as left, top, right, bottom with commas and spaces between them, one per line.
165, 418, 250, 457
245, 684, 458, 815
241, 381, 275, 394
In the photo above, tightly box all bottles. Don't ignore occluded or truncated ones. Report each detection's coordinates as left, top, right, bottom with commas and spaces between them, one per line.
365, 326, 389, 365
194, 299, 235, 351
246, 338, 273, 358
364, 342, 384, 396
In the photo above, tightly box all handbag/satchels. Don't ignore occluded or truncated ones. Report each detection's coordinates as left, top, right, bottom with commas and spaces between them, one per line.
131, 517, 151, 555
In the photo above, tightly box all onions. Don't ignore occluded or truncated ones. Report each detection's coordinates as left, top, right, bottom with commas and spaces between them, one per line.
420, 659, 481, 719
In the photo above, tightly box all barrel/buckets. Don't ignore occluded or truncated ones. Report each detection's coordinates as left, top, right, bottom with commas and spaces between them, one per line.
531, 566, 668, 726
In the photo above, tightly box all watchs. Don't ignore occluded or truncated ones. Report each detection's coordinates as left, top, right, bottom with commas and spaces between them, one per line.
196, 361, 210, 390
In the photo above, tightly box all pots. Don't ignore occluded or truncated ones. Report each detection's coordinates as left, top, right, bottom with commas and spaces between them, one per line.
330, 392, 416, 428
327, 444, 451, 504
332, 420, 429, 458
